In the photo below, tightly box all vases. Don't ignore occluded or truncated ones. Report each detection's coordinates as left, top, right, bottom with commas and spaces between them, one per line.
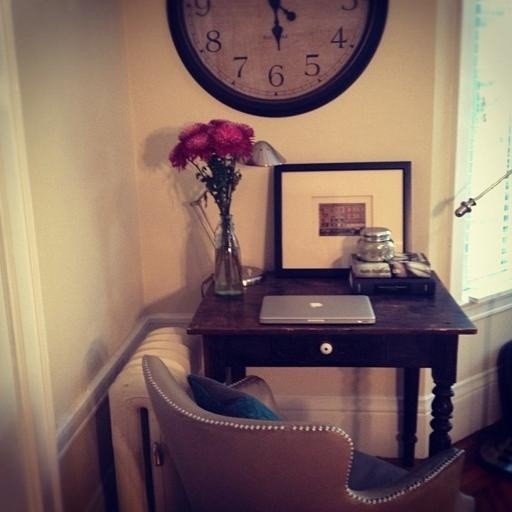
214, 214, 244, 298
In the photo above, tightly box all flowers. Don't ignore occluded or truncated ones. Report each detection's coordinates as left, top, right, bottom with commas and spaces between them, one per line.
170, 120, 254, 285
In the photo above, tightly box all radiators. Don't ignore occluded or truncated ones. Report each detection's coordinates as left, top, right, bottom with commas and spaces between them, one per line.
108, 326, 202, 512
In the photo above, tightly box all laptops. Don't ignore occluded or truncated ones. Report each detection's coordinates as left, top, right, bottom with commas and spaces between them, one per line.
259, 295, 376, 324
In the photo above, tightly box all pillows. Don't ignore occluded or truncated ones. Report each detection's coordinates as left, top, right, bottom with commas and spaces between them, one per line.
188, 373, 280, 421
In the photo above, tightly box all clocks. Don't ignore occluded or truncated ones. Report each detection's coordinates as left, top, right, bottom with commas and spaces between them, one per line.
166, 0, 389, 118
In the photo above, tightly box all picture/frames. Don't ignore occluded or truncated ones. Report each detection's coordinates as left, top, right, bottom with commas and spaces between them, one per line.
274, 161, 411, 279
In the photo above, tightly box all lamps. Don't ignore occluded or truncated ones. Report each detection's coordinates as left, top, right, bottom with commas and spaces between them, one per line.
190, 141, 286, 288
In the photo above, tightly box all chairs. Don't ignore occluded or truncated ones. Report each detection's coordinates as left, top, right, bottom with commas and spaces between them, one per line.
142, 355, 476, 512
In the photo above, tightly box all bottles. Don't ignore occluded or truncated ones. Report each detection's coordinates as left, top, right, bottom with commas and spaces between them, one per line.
355, 227, 394, 262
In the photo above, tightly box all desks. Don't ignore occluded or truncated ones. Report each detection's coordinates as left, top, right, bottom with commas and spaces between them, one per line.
186, 271, 478, 468
